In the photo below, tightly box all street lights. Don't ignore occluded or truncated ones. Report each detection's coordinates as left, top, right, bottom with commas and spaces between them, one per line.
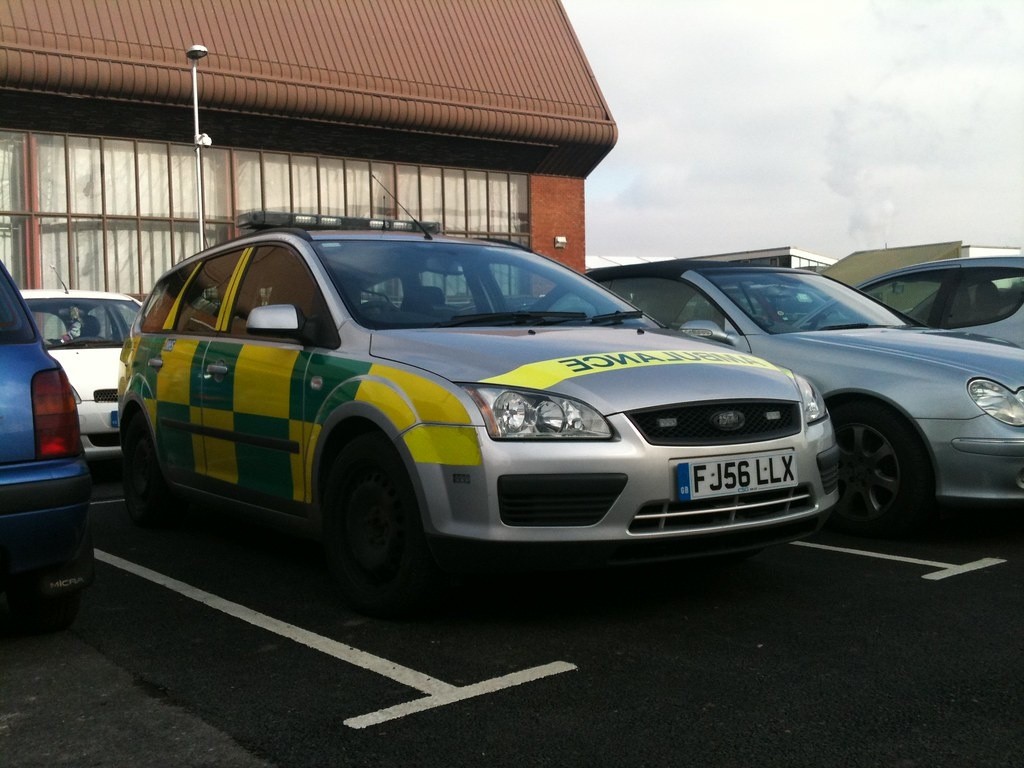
184, 43, 213, 253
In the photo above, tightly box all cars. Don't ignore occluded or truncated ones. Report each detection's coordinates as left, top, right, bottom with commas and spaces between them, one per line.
0, 260, 94, 579
790, 252, 1024, 351
527, 263, 1024, 540
17, 287, 145, 471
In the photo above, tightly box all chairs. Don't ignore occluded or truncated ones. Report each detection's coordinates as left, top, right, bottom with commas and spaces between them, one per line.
398, 286, 458, 325
953, 280, 1006, 328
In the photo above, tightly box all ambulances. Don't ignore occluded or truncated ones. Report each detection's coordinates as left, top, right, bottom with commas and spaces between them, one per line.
114, 210, 841, 626
